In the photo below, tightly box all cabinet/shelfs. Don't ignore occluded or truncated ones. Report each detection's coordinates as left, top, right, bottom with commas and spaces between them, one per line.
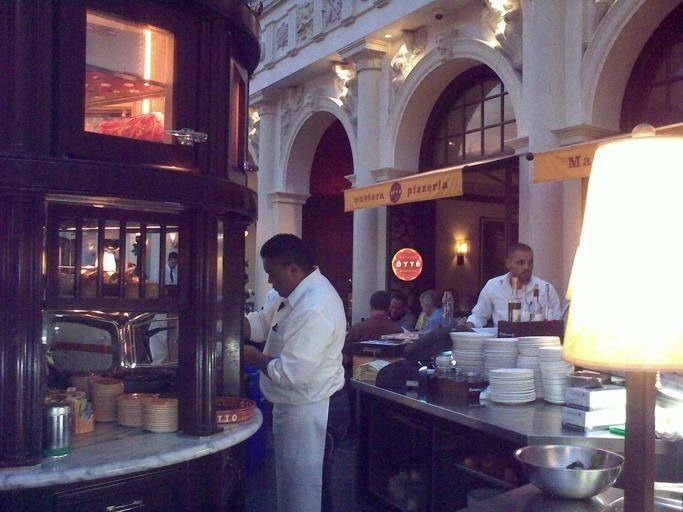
0, 441, 251, 512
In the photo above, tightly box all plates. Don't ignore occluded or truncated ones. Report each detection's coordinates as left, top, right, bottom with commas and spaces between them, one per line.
448, 331, 576, 405
68, 374, 180, 434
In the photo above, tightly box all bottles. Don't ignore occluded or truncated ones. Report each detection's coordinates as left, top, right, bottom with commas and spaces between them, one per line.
42, 403, 70, 457
442, 290, 454, 321
508, 276, 555, 323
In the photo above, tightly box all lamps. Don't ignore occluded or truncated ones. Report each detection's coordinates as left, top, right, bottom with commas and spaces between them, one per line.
455, 241, 469, 265
559, 125, 683, 510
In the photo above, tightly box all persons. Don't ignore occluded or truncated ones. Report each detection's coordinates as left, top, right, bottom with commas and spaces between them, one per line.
419, 289, 456, 334
388, 292, 416, 330
458, 244, 562, 330
345, 288, 405, 358
214, 232, 351, 509
164, 251, 177, 286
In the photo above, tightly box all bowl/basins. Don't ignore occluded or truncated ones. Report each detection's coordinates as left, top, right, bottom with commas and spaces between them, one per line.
569, 372, 611, 386
513, 443, 625, 502
213, 394, 257, 424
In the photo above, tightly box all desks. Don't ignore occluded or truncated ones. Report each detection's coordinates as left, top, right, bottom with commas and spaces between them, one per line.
458, 478, 639, 512
